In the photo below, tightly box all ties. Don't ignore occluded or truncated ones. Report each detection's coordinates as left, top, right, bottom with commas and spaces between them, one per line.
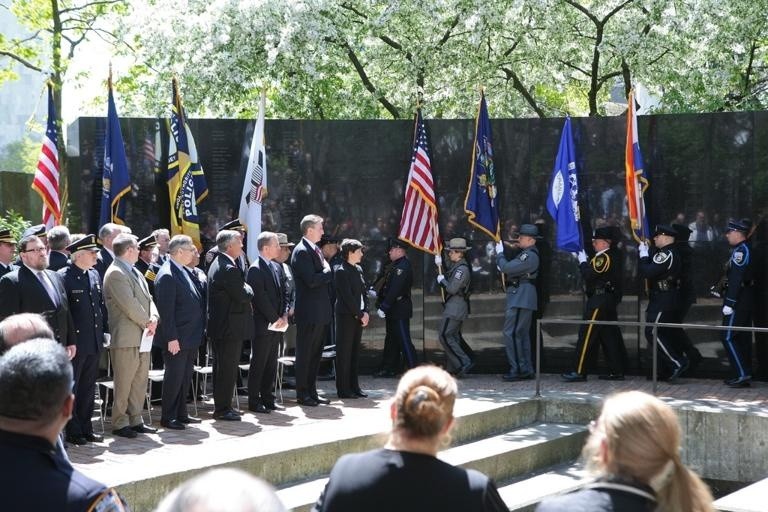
38, 272, 59, 308
316, 247, 326, 266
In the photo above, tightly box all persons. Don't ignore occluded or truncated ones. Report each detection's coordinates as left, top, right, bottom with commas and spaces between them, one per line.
153, 467, 285, 511
495, 224, 538, 381
1, 313, 128, 511
375, 238, 418, 378
560, 228, 628, 381
639, 225, 690, 382
711, 222, 754, 388
314, 365, 510, 511
437, 237, 475, 378
536, 390, 718, 511
325, 202, 767, 298
0, 214, 371, 450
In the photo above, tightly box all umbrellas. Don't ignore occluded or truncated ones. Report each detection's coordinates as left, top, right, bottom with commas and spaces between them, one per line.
237, 91, 268, 268
464, 96, 503, 244
153, 77, 209, 255
100, 75, 134, 241
398, 108, 444, 255
625, 92, 652, 248
30, 85, 62, 231
545, 117, 585, 255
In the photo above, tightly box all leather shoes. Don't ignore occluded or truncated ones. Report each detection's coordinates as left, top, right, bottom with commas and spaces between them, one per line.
374, 368, 398, 378
70, 390, 367, 444
723, 375, 754, 388
560, 371, 626, 383
501, 369, 537, 382
454, 361, 476, 378
668, 359, 691, 381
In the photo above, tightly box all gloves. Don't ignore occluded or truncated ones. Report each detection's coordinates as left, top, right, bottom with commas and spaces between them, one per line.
495, 239, 504, 254
709, 286, 721, 298
369, 290, 377, 297
638, 241, 650, 259
377, 308, 386, 319
436, 274, 445, 284
722, 306, 733, 315
435, 255, 442, 267
571, 248, 589, 264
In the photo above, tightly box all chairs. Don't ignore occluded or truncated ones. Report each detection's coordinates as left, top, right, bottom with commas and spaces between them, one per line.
92, 332, 339, 434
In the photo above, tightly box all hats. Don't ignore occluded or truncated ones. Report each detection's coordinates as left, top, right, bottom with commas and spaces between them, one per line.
388, 237, 409, 250
444, 236, 472, 253
0, 228, 19, 244
218, 218, 249, 233
137, 234, 161, 248
723, 217, 750, 234
513, 223, 545, 241
275, 232, 295, 247
23, 224, 47, 238
64, 234, 100, 254
589, 227, 615, 239
651, 224, 680, 238
